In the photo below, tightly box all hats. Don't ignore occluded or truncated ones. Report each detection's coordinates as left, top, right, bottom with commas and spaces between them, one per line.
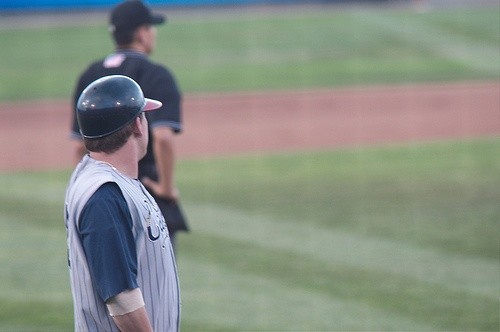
110, 0, 166, 28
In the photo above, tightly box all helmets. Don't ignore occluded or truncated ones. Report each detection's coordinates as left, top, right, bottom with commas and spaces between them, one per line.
77, 74, 162, 138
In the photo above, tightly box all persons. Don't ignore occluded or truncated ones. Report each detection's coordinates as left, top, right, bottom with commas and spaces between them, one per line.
62, 73, 182, 332
71, 0, 192, 265
